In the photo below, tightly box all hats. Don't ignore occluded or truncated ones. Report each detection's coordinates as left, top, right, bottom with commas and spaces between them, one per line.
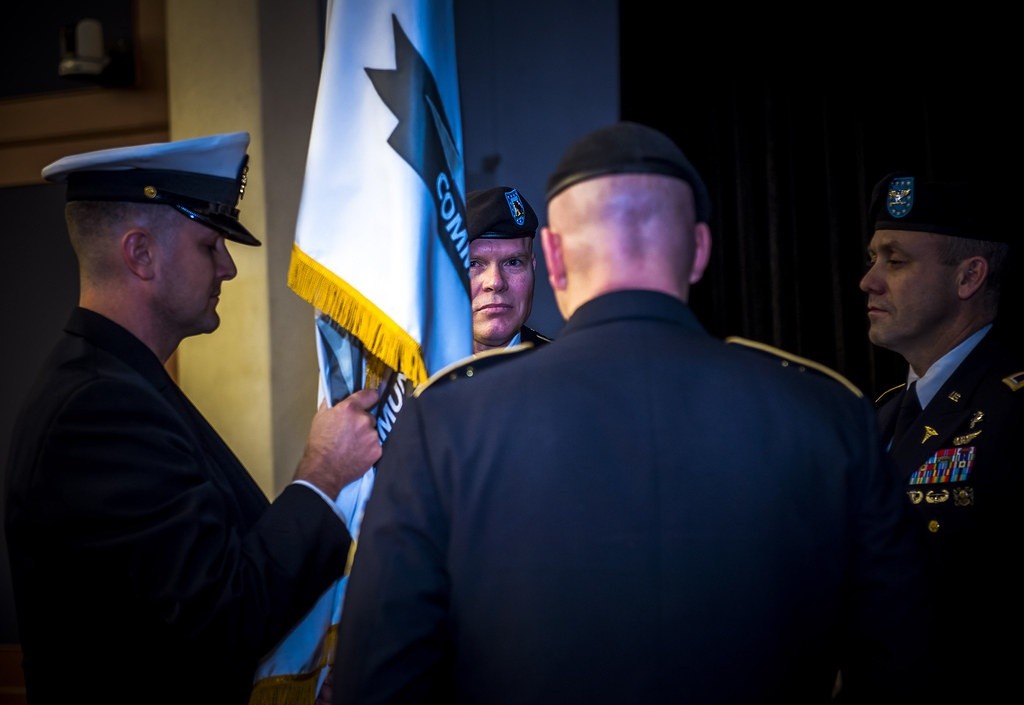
40, 133, 264, 250
869, 161, 1020, 246
545, 121, 710, 224
466, 185, 538, 243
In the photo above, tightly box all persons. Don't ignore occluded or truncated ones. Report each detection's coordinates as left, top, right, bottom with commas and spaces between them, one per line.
857, 168, 1024, 704
312, 122, 920, 704
464, 186, 556, 355
3, 131, 382, 704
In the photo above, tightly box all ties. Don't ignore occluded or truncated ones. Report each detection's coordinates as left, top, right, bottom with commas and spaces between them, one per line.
886, 381, 919, 464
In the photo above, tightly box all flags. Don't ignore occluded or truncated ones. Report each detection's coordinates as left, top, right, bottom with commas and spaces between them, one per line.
247, 1, 475, 705
